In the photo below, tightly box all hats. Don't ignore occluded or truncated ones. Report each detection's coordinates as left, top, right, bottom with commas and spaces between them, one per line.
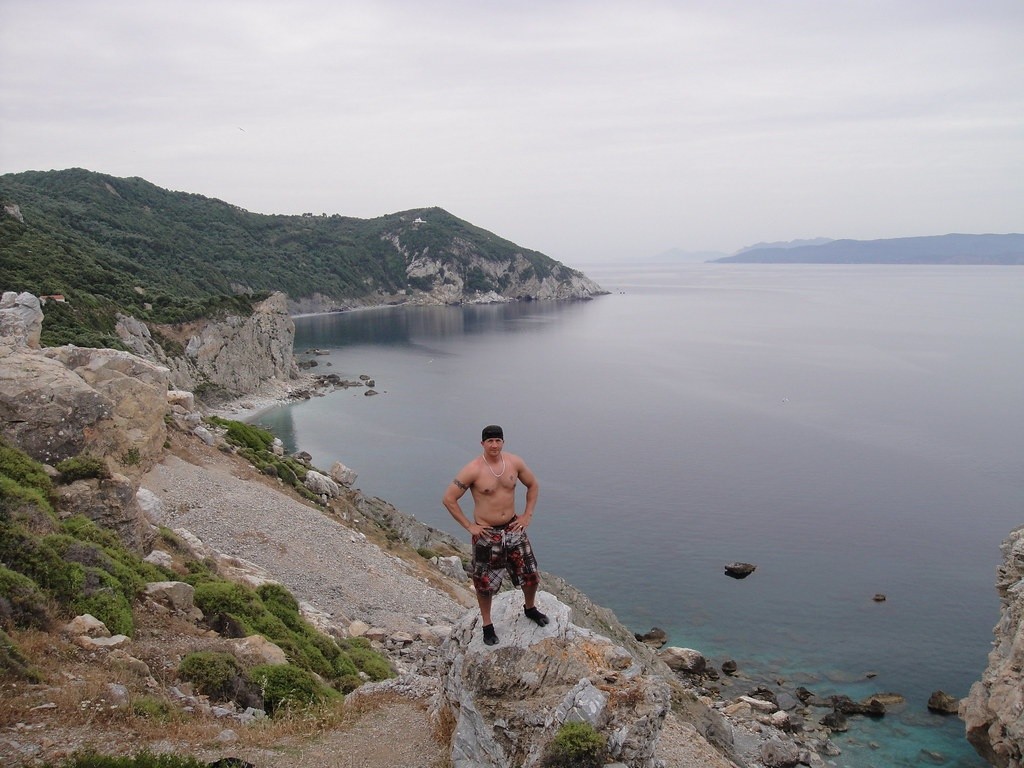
482, 425, 503, 442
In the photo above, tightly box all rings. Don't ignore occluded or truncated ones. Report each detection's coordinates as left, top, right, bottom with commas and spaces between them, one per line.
519, 525, 523, 528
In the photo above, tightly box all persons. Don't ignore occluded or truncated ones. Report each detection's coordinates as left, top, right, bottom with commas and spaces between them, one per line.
442, 425, 550, 646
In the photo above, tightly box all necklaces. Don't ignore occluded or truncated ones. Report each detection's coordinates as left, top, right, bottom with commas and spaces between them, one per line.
482, 452, 506, 478
